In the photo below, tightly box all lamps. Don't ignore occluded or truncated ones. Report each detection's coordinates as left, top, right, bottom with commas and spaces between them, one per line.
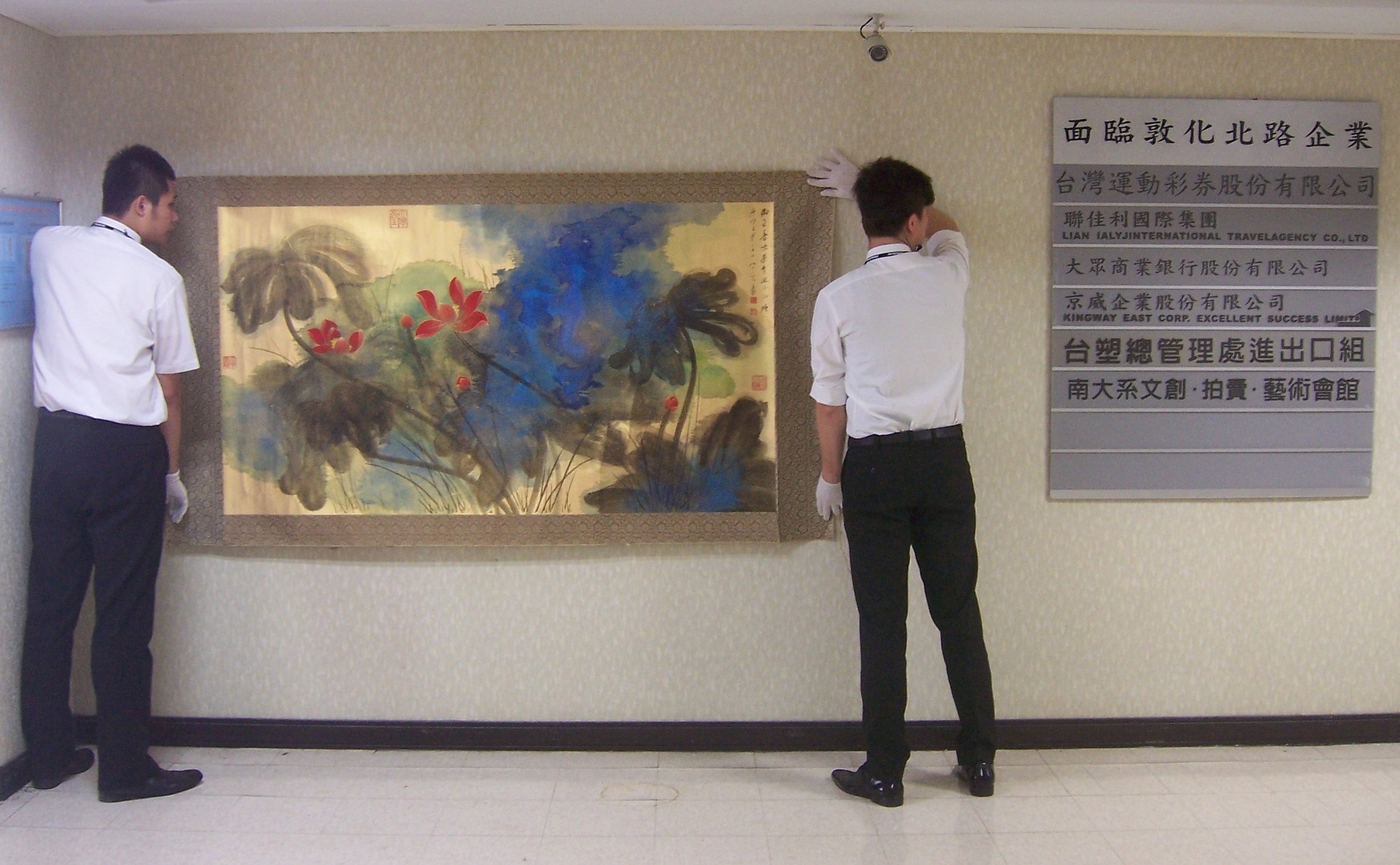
859, 12, 894, 64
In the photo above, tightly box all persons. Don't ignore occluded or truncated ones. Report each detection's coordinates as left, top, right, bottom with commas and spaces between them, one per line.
21, 142, 202, 802
807, 151, 996, 808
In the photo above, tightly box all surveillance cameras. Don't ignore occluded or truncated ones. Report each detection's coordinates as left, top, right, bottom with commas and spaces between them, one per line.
866, 34, 892, 62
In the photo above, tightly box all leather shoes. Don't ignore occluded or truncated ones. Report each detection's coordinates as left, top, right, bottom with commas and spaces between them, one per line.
952, 761, 996, 798
33, 747, 95, 789
832, 765, 903, 807
97, 757, 203, 801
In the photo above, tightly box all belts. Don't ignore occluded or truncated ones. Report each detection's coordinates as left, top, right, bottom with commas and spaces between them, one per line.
39, 407, 102, 421
848, 424, 964, 447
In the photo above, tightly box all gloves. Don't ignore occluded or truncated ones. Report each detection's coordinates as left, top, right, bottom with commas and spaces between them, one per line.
816, 471, 843, 520
806, 148, 862, 201
165, 469, 188, 524
